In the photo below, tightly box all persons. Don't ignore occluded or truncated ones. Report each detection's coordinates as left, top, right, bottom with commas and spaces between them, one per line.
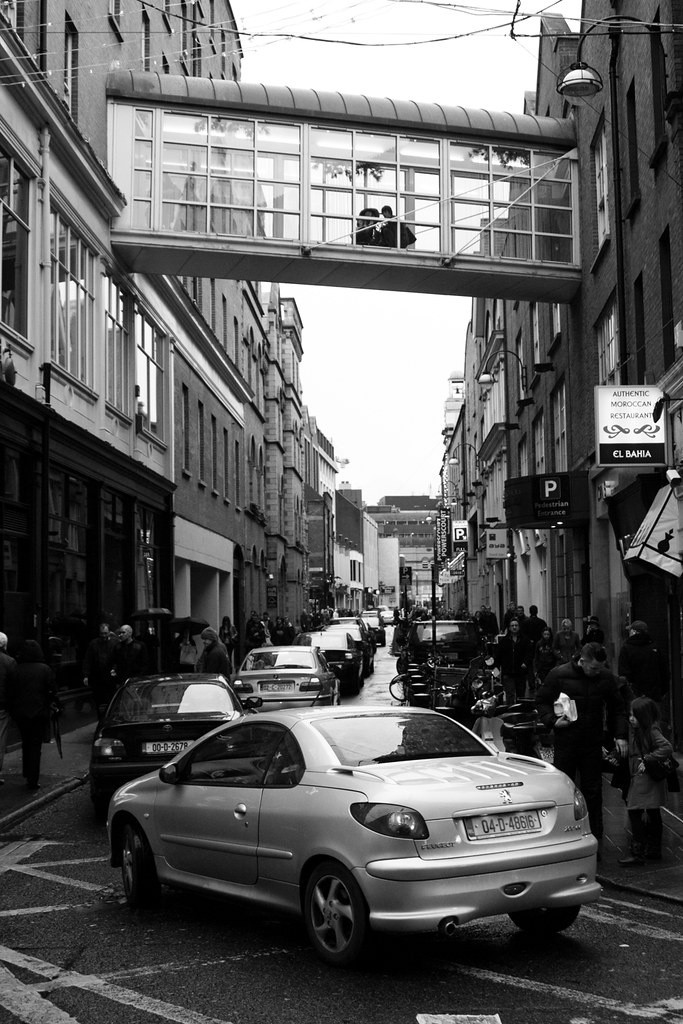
394, 592, 675, 865
0, 601, 381, 790
356, 206, 409, 248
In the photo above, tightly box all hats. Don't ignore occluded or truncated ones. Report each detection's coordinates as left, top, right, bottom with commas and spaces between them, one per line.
586, 616, 600, 624
201, 627, 218, 641
625, 620, 649, 634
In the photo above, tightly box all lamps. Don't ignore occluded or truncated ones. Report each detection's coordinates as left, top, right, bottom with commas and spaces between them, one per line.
448, 442, 480, 467
477, 349, 528, 393
555, 14, 668, 139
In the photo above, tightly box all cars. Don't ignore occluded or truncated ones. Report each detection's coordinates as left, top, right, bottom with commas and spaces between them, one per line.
103, 705, 607, 959
233, 647, 343, 712
89, 668, 264, 823
292, 630, 367, 698
322, 606, 394, 674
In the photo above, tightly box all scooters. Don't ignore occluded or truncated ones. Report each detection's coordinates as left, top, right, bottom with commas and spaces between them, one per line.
434, 649, 546, 762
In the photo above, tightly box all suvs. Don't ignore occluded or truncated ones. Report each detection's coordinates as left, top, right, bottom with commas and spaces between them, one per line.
394, 620, 484, 677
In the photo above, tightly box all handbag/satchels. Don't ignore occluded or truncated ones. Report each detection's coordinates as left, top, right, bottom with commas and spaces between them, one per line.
644, 755, 680, 782
180, 645, 197, 665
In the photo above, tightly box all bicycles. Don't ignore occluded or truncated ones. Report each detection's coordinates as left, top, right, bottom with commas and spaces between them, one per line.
389, 653, 455, 701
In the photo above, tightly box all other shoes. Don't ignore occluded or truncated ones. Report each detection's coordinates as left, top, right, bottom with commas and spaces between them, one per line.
26, 778, 41, 788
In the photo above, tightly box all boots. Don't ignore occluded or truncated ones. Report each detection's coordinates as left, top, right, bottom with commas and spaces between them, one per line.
618, 840, 662, 865
